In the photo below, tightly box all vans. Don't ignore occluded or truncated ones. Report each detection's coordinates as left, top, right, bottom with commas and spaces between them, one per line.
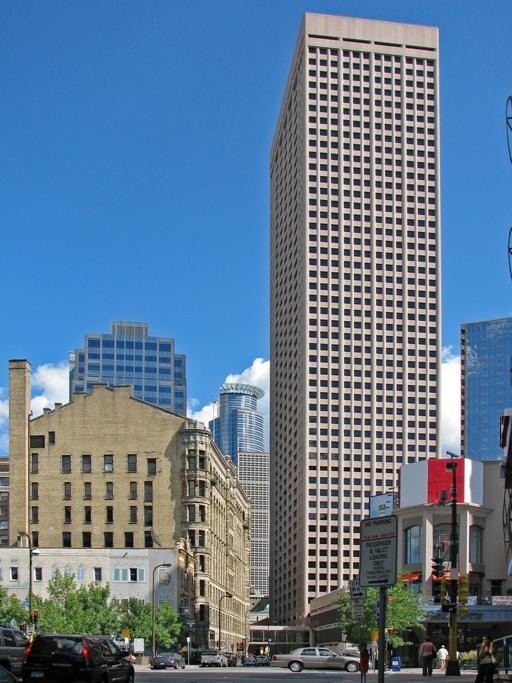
315, 642, 353, 653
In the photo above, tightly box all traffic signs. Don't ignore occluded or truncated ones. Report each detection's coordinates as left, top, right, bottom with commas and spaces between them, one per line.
358, 512, 399, 588
21, 601, 30, 605
351, 597, 365, 618
460, 578, 468, 603
442, 578, 449, 597
349, 579, 368, 600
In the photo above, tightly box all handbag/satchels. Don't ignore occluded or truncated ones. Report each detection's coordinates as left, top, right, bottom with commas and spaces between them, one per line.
491, 655, 498, 664
431, 646, 437, 658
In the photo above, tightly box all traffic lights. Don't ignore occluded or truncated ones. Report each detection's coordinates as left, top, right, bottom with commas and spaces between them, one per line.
431, 556, 442, 574
32, 609, 39, 624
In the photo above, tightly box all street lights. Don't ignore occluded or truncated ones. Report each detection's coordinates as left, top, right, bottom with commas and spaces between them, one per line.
268, 638, 271, 656
19, 528, 35, 635
152, 563, 171, 660
444, 459, 460, 677
217, 591, 232, 649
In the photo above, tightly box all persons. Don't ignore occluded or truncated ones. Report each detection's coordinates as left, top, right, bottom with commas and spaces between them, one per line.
455, 649, 463, 662
419, 635, 437, 676
475, 633, 496, 683
359, 641, 370, 682
436, 644, 449, 672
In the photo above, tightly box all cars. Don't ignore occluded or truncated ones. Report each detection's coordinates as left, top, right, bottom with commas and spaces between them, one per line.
269, 643, 372, 672
224, 650, 271, 669
336, 648, 359, 658
151, 651, 186, 670
0, 664, 20, 682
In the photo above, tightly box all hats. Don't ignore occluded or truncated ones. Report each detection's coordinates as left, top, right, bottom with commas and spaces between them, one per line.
441, 645, 445, 647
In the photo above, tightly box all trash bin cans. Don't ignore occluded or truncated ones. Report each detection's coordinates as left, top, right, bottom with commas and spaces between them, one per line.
392, 656, 401, 671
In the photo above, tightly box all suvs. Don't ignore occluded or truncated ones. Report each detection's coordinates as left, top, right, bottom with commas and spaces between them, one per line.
20, 634, 135, 682
0, 623, 32, 676
200, 647, 228, 667
